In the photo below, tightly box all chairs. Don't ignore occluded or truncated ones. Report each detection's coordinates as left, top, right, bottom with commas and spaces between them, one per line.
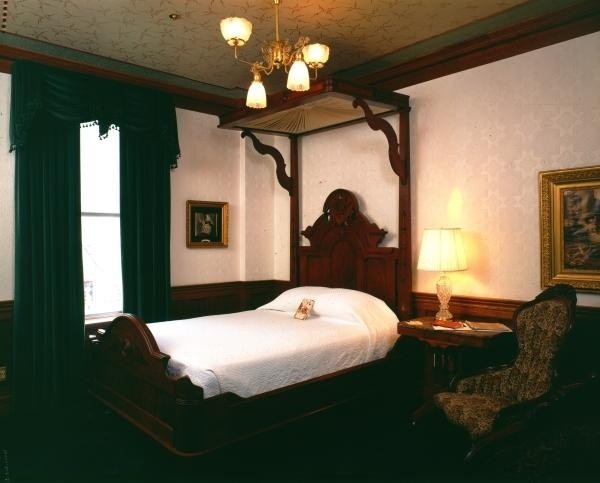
405, 283, 577, 461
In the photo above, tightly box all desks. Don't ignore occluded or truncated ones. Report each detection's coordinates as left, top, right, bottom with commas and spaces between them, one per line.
396, 314, 515, 400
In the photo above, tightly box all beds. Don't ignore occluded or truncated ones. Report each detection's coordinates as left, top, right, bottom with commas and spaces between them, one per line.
82, 78, 414, 457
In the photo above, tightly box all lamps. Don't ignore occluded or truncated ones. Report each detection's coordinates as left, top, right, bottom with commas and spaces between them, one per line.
219, 1, 332, 110
415, 227, 468, 326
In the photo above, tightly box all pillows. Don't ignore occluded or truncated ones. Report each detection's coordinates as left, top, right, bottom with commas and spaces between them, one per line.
313, 289, 399, 329
257, 282, 326, 317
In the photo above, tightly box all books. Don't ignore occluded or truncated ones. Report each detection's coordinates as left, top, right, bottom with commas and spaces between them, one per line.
432, 319, 464, 330
293, 298, 316, 321
465, 320, 508, 331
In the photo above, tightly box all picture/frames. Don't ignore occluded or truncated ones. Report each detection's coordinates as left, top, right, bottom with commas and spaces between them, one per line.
184, 199, 229, 250
539, 165, 600, 297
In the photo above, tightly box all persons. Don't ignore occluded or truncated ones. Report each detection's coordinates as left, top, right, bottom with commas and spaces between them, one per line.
198, 212, 207, 236
204, 211, 214, 230
